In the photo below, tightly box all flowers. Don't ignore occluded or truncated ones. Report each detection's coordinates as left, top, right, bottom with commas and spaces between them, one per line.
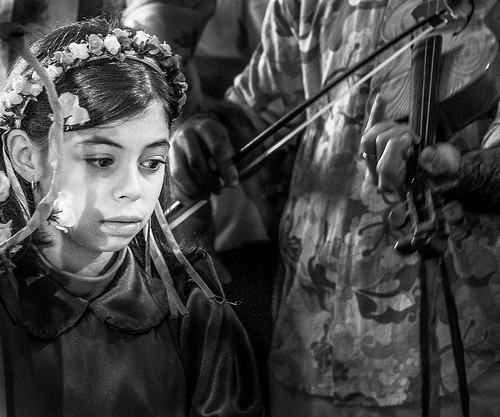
0, 28, 188, 203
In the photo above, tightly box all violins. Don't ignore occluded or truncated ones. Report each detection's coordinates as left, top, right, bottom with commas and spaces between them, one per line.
368, 0, 500, 262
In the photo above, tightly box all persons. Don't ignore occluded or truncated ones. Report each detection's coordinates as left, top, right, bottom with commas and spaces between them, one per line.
0, 18, 264, 417
167, 0, 500, 417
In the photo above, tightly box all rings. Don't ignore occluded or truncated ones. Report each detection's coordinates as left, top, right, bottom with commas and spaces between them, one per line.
361, 152, 377, 162
